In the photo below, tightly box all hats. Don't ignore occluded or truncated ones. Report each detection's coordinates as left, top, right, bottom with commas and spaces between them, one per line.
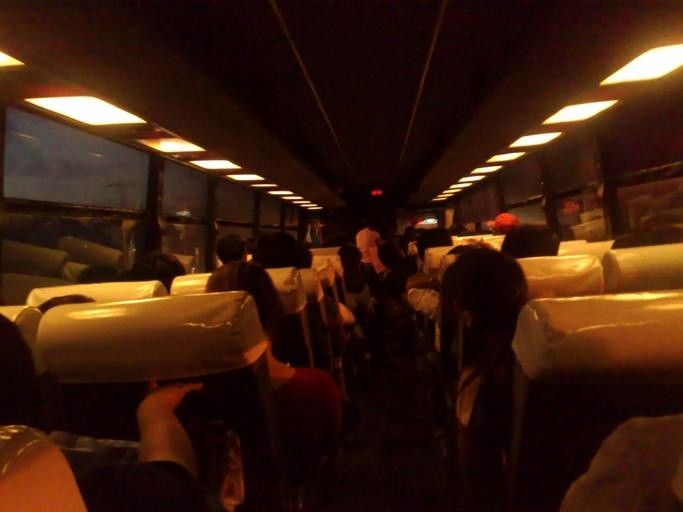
486, 213, 519, 228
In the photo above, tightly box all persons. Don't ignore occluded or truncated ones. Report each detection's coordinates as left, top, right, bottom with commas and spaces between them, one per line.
2, 209, 682, 512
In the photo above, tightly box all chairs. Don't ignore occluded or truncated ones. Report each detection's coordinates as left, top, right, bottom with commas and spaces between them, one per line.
405, 232, 683, 510
0, 246, 345, 511
